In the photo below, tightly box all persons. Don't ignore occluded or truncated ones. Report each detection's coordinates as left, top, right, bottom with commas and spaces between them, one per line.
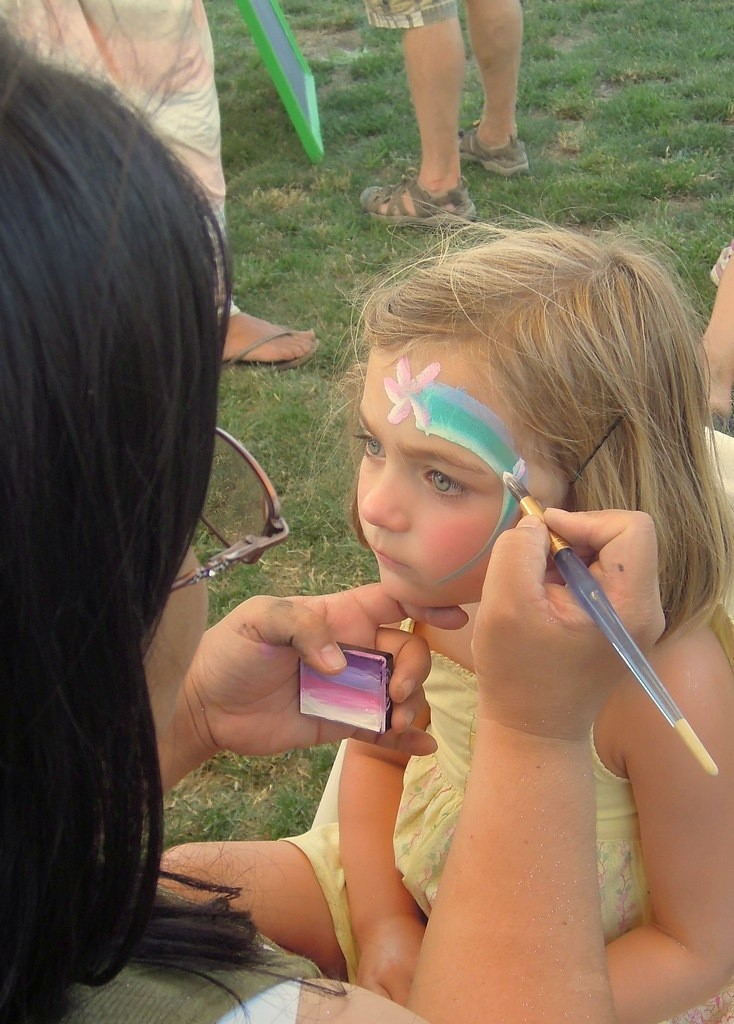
0, 16, 669, 1023
698, 237, 733, 419
354, 0, 530, 224
148, 200, 734, 1023
0, 2, 322, 372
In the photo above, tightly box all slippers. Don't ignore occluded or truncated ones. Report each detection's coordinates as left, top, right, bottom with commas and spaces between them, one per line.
225, 324, 320, 375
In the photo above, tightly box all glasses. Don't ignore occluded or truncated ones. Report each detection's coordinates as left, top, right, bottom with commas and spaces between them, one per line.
173, 427, 287, 597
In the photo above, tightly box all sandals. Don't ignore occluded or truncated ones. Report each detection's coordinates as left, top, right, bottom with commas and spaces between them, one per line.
360, 168, 475, 227
457, 125, 530, 176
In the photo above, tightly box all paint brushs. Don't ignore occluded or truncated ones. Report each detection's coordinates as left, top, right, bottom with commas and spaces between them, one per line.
500, 470, 724, 778
